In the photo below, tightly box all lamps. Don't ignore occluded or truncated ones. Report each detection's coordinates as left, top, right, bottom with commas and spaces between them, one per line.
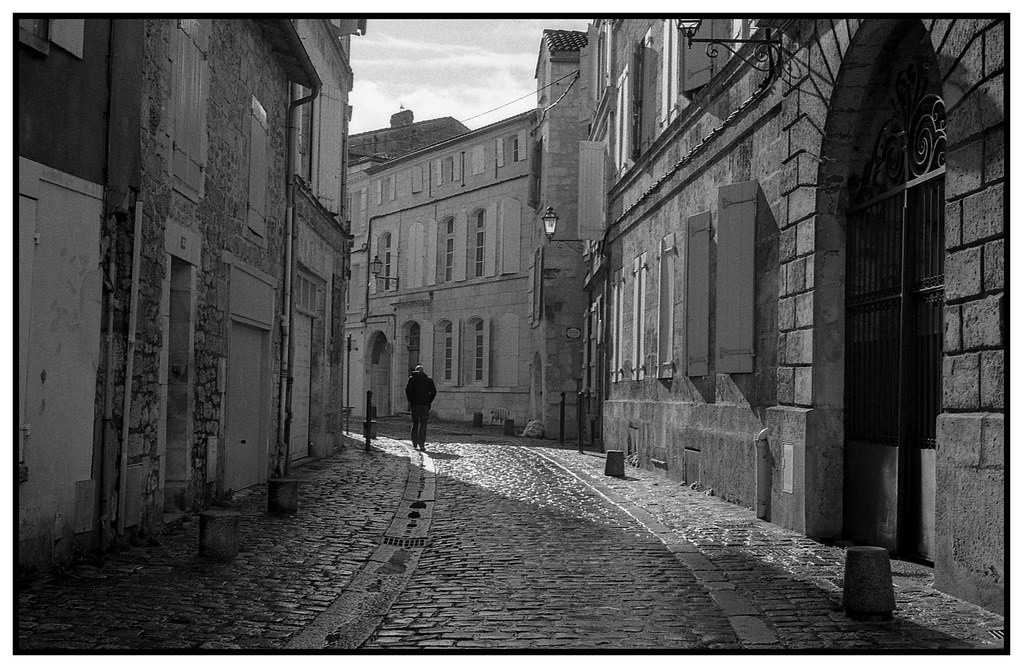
369, 256, 399, 292
541, 206, 583, 256
676, 19, 783, 80
383, 343, 393, 357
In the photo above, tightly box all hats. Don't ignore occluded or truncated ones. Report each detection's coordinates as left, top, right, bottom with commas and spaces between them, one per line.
415, 366, 423, 372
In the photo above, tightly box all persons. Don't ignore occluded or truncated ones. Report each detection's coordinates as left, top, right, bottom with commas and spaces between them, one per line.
405, 366, 437, 450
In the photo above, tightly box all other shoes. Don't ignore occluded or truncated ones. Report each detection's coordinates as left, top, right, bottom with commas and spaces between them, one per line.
412, 445, 417, 450
419, 444, 424, 450
